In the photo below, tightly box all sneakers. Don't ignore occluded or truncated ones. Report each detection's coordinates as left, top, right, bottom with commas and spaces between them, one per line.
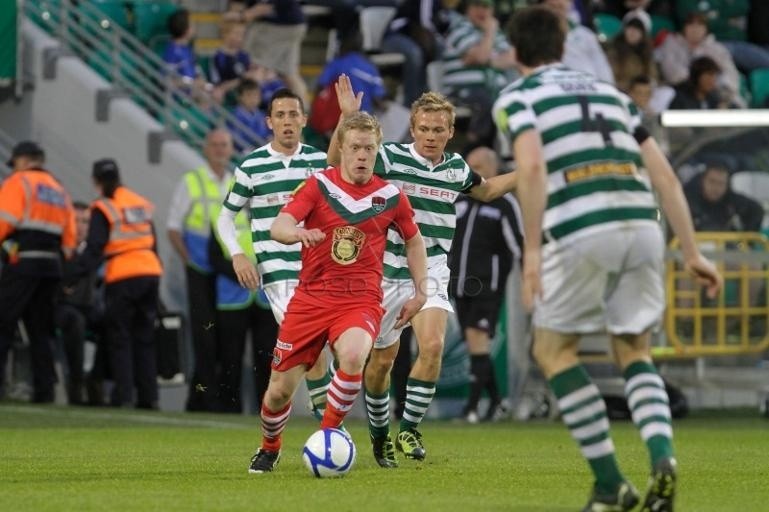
372, 431, 399, 468
639, 457, 677, 512
395, 427, 427, 461
247, 446, 281, 475
579, 480, 641, 512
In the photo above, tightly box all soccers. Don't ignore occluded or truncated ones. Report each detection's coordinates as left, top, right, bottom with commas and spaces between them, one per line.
302, 429, 356, 478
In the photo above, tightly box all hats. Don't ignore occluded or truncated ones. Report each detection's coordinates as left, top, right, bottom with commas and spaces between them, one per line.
8, 142, 42, 164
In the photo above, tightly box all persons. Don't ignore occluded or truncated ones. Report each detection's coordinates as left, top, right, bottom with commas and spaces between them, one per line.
215, 88, 353, 444
208, 175, 278, 415
313, 39, 385, 115
229, 80, 273, 154
247, 110, 428, 474
86, 160, 165, 409
446, 146, 524, 428
670, 56, 742, 110
440, 0, 516, 145
226, 0, 309, 106
158, 10, 207, 105
165, 127, 238, 413
47, 200, 109, 408
661, 11, 747, 108
0, 142, 77, 406
489, 6, 722, 512
679, 159, 764, 236
364, 0, 446, 109
207, 20, 284, 105
536, 0, 617, 87
325, 72, 515, 470
624, 77, 673, 159
602, 7, 658, 91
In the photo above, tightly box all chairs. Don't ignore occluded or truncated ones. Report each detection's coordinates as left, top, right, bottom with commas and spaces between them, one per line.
730, 169, 769, 230
